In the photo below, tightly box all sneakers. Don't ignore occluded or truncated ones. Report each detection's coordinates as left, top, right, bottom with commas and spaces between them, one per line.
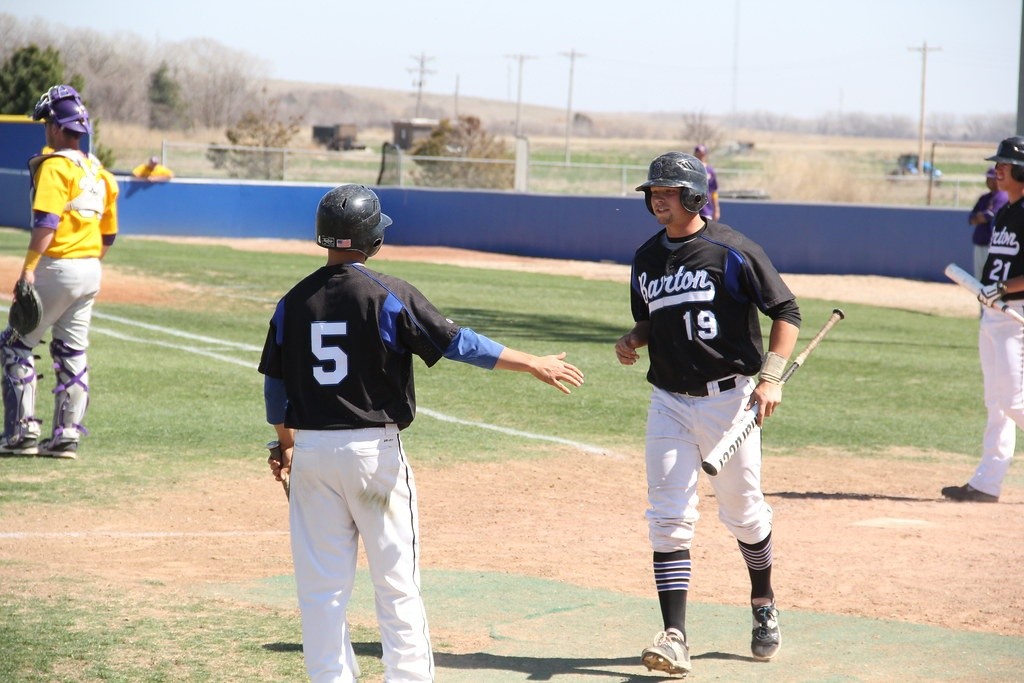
941, 482, 999, 503
751, 595, 781, 659
0, 432, 39, 455
38, 440, 77, 459
641, 631, 691, 674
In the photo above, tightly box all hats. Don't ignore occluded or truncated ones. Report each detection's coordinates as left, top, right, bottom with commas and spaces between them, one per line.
983, 167, 996, 178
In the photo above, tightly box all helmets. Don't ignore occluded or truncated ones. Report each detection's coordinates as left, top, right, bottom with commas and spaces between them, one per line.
985, 136, 1024, 168
33, 85, 90, 134
635, 151, 709, 216
315, 184, 393, 257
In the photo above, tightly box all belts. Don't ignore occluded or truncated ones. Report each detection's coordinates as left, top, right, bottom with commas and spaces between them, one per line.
680, 376, 737, 396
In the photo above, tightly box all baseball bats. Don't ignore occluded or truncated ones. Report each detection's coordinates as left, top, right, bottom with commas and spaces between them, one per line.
701, 307, 845, 476
942, 263, 1024, 326
265, 440, 289, 502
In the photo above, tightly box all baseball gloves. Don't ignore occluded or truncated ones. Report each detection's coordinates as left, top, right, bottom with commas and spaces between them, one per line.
8, 279, 43, 338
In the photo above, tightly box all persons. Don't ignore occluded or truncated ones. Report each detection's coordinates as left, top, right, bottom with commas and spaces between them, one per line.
694, 145, 720, 221
0, 86, 119, 459
968, 170, 1010, 315
132, 157, 171, 182
258, 184, 584, 682
616, 152, 802, 676
941, 137, 1024, 503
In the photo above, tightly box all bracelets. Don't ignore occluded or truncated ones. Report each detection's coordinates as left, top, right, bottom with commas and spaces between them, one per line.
759, 352, 787, 385
